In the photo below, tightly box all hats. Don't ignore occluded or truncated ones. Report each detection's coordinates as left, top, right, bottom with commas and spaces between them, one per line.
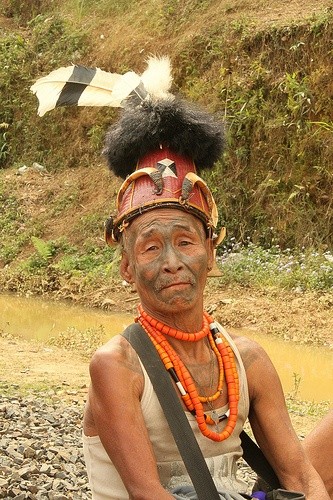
33, 56, 228, 246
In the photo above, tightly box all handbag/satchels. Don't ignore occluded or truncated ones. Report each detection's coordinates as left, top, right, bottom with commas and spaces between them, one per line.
122, 323, 307, 500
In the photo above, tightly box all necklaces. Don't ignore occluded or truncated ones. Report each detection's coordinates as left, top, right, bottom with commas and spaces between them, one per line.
136, 301, 241, 442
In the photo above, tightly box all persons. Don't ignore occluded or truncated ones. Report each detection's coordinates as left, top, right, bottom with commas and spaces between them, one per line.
79, 172, 333, 500
